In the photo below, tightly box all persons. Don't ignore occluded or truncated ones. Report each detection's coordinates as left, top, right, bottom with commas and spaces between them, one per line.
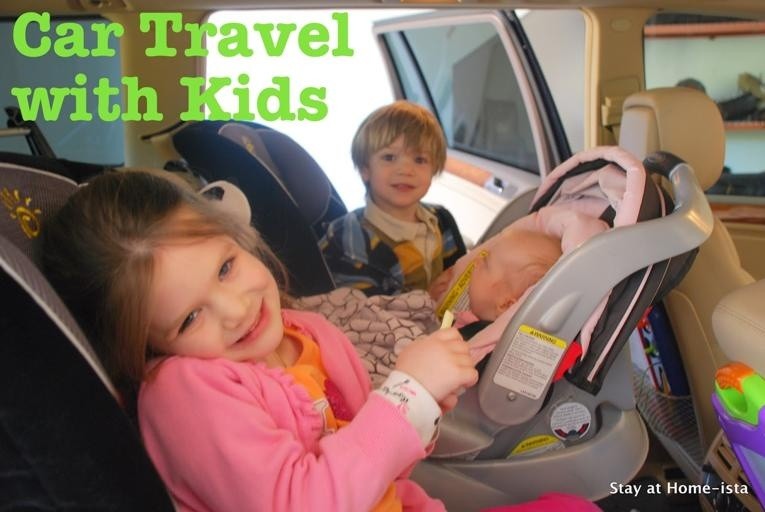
457, 229, 562, 383
317, 100, 468, 299
41, 169, 604, 512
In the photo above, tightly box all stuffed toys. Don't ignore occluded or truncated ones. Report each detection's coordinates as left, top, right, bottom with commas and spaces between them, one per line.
712, 363, 765, 512
625, 300, 695, 439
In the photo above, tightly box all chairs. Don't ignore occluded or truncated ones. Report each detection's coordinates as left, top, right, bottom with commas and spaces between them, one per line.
0, 152, 175, 510
617, 85, 760, 462
699, 279, 764, 508
171, 116, 348, 299
